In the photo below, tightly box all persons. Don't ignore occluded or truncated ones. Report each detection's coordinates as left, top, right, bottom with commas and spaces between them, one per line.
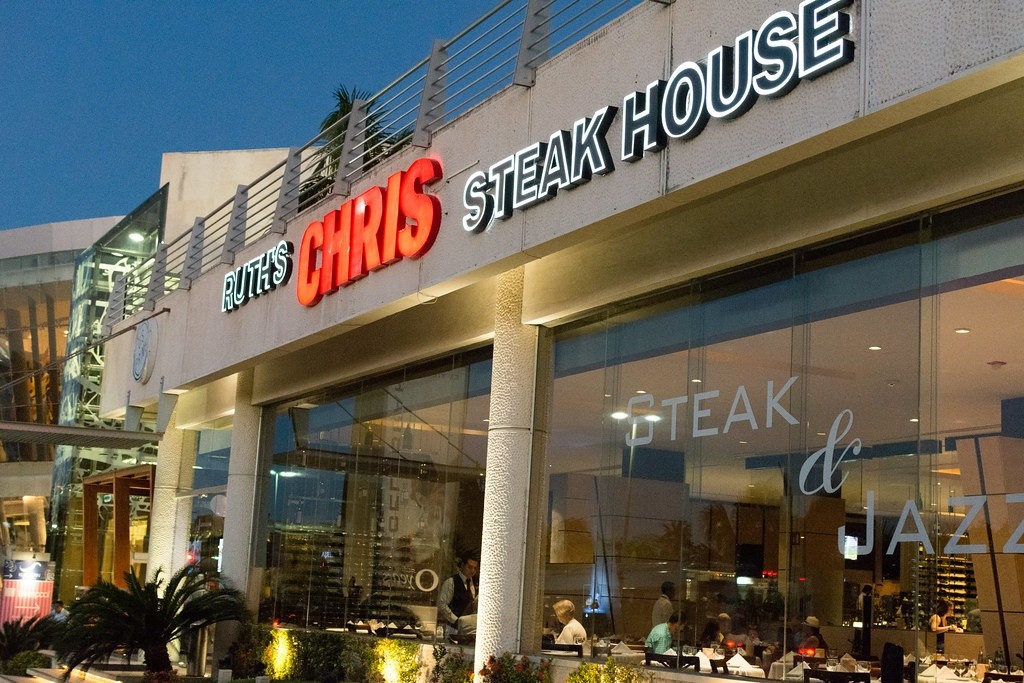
553, 600, 587, 645
928, 602, 957, 633
651, 581, 676, 627
965, 596, 983, 632
438, 554, 478, 634
646, 610, 688, 654
701, 612, 828, 653
855, 584, 872, 610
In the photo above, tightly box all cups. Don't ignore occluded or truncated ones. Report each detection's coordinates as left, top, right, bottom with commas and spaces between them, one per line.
684, 646, 697, 655
942, 654, 966, 678
826, 659, 871, 673
578, 637, 585, 644
710, 644, 724, 655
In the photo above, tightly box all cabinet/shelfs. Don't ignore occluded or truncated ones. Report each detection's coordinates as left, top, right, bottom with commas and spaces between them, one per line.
912, 555, 977, 618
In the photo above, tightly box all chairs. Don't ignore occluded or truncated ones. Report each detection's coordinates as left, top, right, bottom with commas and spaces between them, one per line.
803, 669, 823, 683
448, 634, 476, 646
593, 647, 612, 658
346, 623, 372, 634
644, 652, 700, 671
709, 659, 729, 675
542, 644, 583, 659
984, 672, 1023, 683
821, 670, 870, 683
793, 655, 826, 668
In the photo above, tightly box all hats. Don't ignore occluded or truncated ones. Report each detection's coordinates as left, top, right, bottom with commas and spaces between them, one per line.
797, 616, 820, 630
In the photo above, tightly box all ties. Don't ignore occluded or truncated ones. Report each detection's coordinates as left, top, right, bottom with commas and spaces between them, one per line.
466, 578, 473, 599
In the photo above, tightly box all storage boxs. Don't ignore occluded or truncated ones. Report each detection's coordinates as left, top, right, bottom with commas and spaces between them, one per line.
976, 664, 990, 679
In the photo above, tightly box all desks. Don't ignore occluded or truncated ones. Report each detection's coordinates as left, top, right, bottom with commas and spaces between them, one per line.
329, 628, 444, 643
543, 644, 982, 683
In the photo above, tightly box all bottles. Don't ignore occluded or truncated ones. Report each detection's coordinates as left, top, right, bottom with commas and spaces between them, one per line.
437, 625, 443, 637
979, 646, 1004, 672
